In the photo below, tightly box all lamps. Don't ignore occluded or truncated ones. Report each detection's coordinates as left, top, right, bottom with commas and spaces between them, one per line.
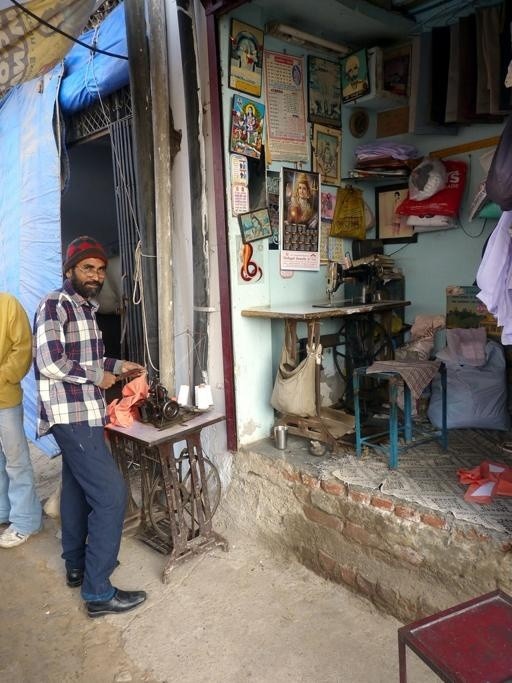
266, 21, 353, 59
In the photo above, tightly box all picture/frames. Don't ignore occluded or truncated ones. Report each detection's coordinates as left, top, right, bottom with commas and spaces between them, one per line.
228, 17, 265, 98
375, 182, 418, 245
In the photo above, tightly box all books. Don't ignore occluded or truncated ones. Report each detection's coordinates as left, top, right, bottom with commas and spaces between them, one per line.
352, 254, 395, 279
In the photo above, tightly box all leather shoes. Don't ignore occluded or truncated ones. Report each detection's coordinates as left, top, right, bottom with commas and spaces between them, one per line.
86, 589, 146, 618
67, 560, 119, 588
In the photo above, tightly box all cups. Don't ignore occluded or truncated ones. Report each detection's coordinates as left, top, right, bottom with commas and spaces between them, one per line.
273, 426, 288, 450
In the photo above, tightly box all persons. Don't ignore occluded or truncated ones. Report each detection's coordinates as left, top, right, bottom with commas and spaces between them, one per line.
32, 236, 146, 618
342, 56, 368, 99
0, 292, 41, 548
392, 192, 400, 236
287, 174, 318, 224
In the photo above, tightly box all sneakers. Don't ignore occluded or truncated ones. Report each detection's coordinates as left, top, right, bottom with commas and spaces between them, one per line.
0, 521, 44, 547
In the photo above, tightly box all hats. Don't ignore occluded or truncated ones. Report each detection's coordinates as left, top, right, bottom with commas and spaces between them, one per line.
61, 236, 109, 277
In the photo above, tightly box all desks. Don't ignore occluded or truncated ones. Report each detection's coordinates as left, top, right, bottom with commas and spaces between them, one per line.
242, 298, 411, 458
104, 408, 231, 582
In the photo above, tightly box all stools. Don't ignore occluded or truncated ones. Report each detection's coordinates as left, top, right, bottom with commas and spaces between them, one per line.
398, 587, 512, 682
353, 361, 447, 469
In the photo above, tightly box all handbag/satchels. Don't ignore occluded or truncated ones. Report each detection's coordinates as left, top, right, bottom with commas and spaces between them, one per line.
269, 348, 319, 419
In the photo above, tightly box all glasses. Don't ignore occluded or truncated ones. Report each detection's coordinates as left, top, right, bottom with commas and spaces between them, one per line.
77, 266, 107, 278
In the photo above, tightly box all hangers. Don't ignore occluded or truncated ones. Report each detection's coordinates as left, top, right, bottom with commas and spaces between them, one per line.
407, 0, 508, 36
